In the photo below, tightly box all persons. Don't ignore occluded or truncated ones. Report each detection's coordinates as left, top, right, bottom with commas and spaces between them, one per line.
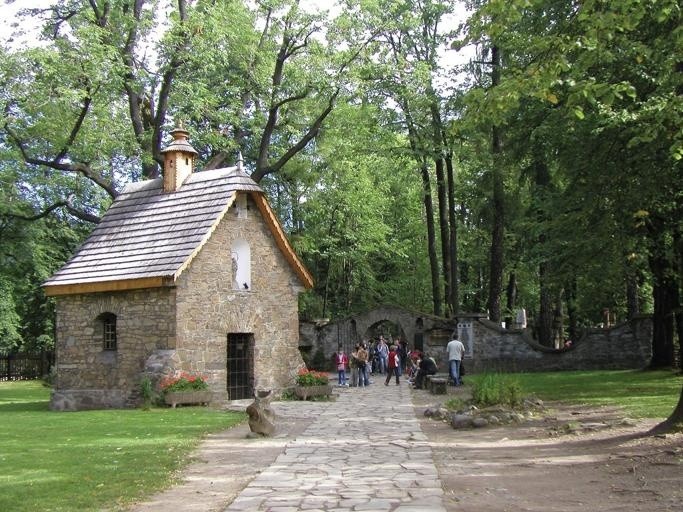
445, 334, 466, 386
461, 294, 469, 313
516, 304, 527, 330
335, 335, 438, 390
563, 340, 570, 352
492, 295, 502, 326
472, 291, 481, 313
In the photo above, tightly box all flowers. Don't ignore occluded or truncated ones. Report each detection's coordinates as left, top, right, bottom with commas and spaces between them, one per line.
297, 367, 329, 386
159, 370, 209, 392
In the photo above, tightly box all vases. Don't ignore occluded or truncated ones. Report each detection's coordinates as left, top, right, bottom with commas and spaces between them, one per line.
165, 390, 212, 408
295, 386, 332, 401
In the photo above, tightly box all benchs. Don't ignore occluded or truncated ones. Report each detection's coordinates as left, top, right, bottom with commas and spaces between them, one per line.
422, 375, 448, 394
